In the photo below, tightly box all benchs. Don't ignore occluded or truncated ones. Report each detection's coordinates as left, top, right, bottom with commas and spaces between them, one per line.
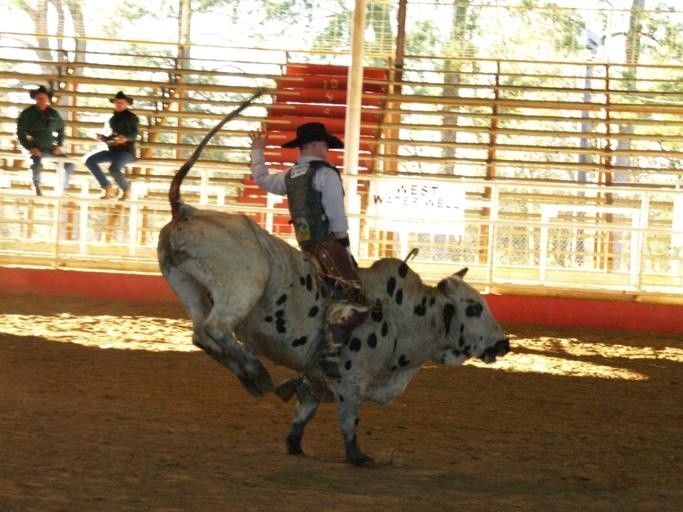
0, 34, 683, 277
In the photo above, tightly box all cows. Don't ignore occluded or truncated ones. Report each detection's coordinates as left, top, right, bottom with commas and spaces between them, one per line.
157, 91, 511, 467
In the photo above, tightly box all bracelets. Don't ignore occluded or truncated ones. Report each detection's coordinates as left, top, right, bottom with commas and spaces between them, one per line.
336, 236, 351, 247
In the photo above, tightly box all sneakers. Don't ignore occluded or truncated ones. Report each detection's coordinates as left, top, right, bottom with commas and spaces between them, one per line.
35, 184, 43, 196
319, 347, 343, 384
100, 181, 132, 201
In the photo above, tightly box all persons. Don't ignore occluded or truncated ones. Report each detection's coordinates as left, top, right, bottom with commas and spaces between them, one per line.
86, 91, 142, 200
248, 119, 370, 383
17, 85, 73, 197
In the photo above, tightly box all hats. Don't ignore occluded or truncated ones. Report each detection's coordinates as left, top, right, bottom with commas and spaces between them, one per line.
109, 91, 134, 105
282, 122, 344, 149
29, 85, 54, 100
